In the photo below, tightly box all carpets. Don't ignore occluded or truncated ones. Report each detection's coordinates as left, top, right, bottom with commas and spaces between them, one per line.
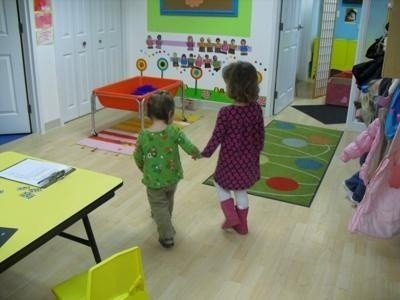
289, 104, 348, 125
77, 109, 204, 158
202, 119, 344, 209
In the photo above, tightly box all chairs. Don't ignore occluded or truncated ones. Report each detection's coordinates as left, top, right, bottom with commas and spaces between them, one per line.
50, 246, 148, 300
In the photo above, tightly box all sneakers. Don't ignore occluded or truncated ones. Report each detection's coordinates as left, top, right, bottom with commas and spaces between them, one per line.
232, 204, 250, 235
158, 237, 174, 248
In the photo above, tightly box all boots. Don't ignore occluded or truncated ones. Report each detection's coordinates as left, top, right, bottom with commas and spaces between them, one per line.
220, 198, 241, 229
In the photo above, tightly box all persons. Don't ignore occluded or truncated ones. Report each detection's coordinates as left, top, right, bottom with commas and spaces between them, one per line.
200, 62, 264, 237
132, 89, 199, 248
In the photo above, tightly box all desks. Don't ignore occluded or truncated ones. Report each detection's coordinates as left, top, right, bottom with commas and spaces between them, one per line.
0, 150, 123, 273
